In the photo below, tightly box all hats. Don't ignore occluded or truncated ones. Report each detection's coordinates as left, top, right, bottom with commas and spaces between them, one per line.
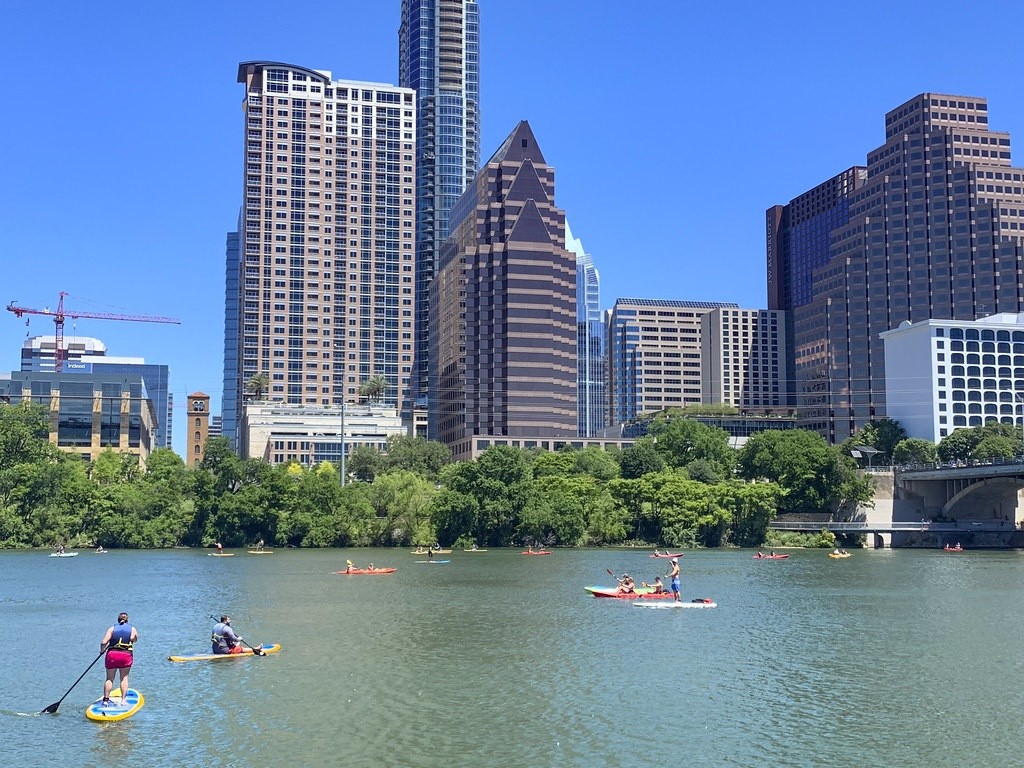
654, 577, 660, 580
622, 573, 629, 577
672, 558, 678, 563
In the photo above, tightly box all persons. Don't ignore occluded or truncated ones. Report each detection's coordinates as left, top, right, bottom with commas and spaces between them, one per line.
654, 549, 660, 557
99, 612, 138, 708
770, 549, 777, 557
211, 615, 263, 655
256, 538, 264, 551
367, 562, 378, 571
434, 542, 441, 552
665, 549, 670, 555
758, 550, 768, 559
616, 573, 636, 595
428, 549, 433, 561
214, 542, 223, 554
664, 556, 682, 603
642, 576, 663, 595
342, 559, 353, 572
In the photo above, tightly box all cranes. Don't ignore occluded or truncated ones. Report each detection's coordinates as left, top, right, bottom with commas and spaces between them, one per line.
5, 289, 182, 373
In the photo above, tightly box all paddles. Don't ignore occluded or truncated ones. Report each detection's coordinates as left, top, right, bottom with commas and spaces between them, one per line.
346, 560, 358, 568
606, 568, 622, 584
41, 643, 110, 714
642, 581, 652, 587
665, 560, 672, 578
210, 614, 266, 656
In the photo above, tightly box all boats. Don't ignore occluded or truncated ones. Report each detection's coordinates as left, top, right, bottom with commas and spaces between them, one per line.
943, 547, 964, 552
828, 553, 853, 559
648, 553, 684, 558
752, 554, 790, 560
584, 585, 675, 599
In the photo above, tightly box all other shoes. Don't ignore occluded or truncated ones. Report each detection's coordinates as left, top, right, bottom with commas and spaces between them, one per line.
102, 701, 108, 707
